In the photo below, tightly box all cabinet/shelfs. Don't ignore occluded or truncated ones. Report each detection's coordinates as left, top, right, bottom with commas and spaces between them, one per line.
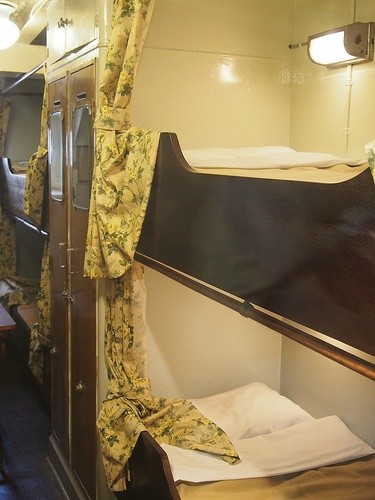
46, 56, 98, 500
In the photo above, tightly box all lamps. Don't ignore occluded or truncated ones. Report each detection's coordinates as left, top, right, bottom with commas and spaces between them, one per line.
0, 2, 20, 51
307, 21, 374, 70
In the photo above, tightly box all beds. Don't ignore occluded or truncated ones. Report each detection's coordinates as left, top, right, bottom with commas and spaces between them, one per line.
1, 157, 48, 237
12, 304, 51, 414
133, 132, 375, 382
123, 383, 375, 500
